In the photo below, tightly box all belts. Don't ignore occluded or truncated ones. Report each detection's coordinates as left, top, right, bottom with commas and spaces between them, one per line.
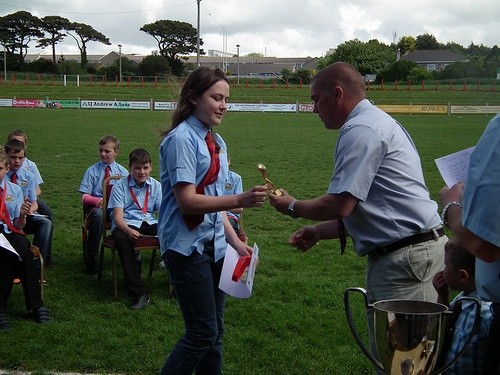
368, 228, 444, 258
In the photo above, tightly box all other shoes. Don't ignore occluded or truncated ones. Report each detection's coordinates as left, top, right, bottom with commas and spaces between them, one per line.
46, 256, 52, 265
38, 277, 48, 287
136, 260, 142, 267
0, 314, 10, 329
131, 294, 150, 309
169, 292, 177, 299
12, 271, 22, 285
92, 269, 97, 274
35, 306, 52, 323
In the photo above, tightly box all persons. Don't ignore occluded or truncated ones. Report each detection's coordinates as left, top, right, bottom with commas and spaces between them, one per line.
225, 150, 242, 237
269, 61, 448, 375
439, 114, 500, 375
432, 240, 493, 375
153, 67, 268, 375
3, 139, 51, 286
8, 130, 53, 270
107, 148, 178, 309
79, 136, 141, 275
0, 144, 53, 330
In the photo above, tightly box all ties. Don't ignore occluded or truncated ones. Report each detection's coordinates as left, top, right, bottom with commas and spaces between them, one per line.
338, 219, 347, 255
105, 167, 110, 184
12, 173, 17, 184
0, 187, 25, 234
181, 133, 220, 232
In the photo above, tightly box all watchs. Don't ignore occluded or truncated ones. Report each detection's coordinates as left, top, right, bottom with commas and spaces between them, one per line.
442, 200, 463, 229
288, 198, 300, 219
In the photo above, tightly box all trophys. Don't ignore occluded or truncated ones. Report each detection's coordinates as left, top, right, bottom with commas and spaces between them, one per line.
344, 287, 482, 375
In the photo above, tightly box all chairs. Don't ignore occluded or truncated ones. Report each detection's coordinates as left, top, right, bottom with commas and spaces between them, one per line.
238, 211, 248, 246
97, 175, 174, 297
